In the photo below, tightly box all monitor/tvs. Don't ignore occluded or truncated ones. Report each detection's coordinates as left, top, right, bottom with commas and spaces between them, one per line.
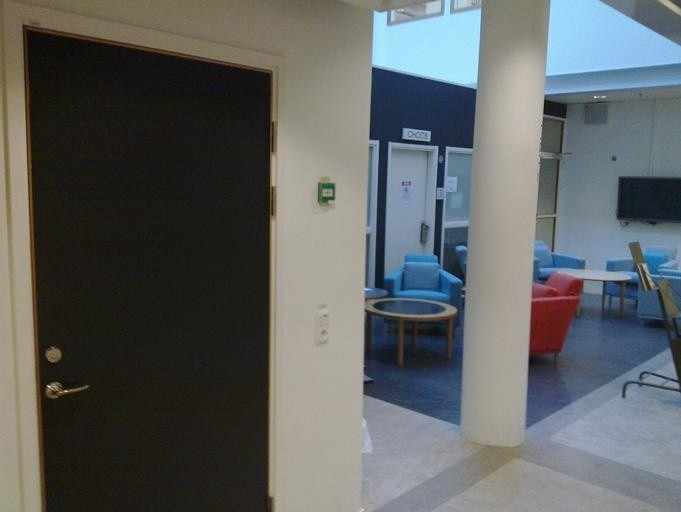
617, 176, 681, 223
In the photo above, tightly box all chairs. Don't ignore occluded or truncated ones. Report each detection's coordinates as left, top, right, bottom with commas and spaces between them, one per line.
384, 240, 681, 362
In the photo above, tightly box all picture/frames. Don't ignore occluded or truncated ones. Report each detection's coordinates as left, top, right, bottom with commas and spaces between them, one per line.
387, 0, 445, 26
450, 0, 481, 13
